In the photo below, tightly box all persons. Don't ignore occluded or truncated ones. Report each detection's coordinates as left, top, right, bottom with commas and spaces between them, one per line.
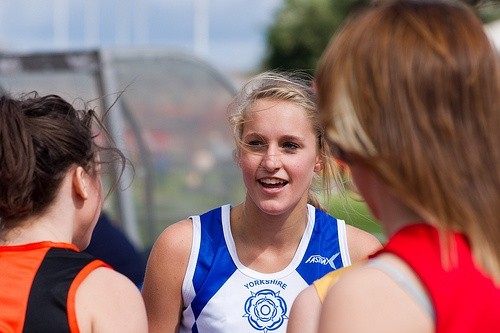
285, 255, 358, 333
311, 2, 500, 332
1, 85, 151, 333
142, 68, 383, 333
